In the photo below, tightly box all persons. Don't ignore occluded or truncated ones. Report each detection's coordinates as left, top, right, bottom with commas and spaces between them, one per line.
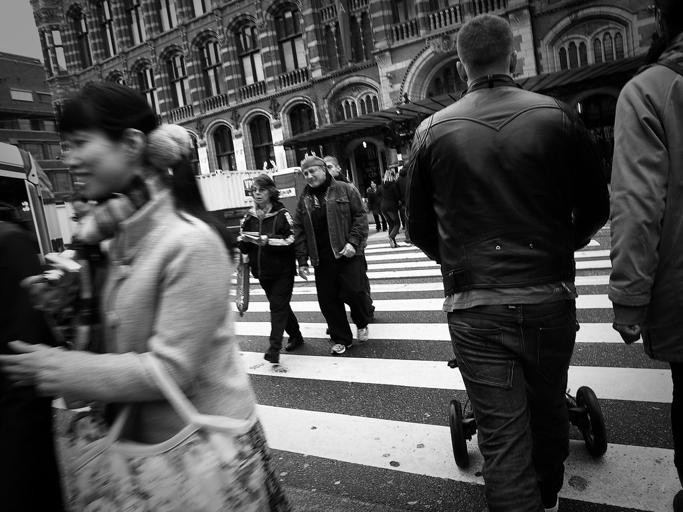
367, 169, 407, 247
607, 0, 683, 512
401, 15, 610, 512
240, 157, 375, 366
0, 82, 292, 512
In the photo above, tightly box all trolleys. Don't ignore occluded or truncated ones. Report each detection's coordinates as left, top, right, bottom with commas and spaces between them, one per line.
444, 320, 608, 470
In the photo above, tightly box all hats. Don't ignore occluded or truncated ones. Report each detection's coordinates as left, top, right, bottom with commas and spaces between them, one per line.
299, 154, 326, 169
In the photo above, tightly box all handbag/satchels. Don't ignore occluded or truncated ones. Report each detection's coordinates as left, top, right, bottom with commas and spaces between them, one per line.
61, 352, 270, 511
235, 247, 252, 317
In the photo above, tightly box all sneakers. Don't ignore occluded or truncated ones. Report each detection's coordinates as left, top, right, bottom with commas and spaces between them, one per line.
285, 340, 304, 352
356, 326, 370, 344
330, 342, 353, 356
376, 228, 414, 248
264, 350, 280, 364
